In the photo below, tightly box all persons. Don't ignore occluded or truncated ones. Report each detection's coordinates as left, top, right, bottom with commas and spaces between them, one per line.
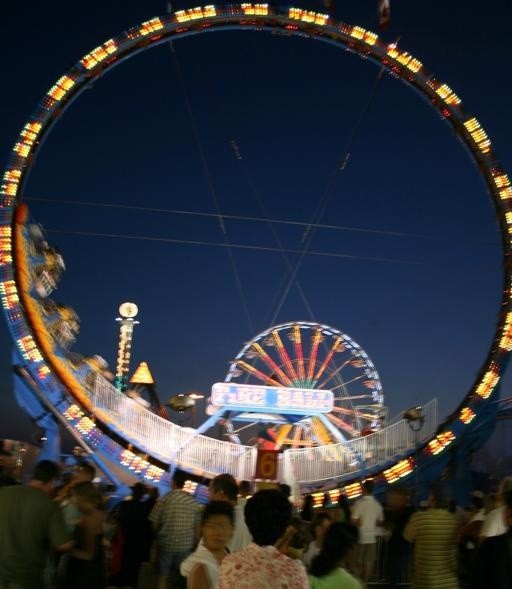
0, 459, 512, 589
